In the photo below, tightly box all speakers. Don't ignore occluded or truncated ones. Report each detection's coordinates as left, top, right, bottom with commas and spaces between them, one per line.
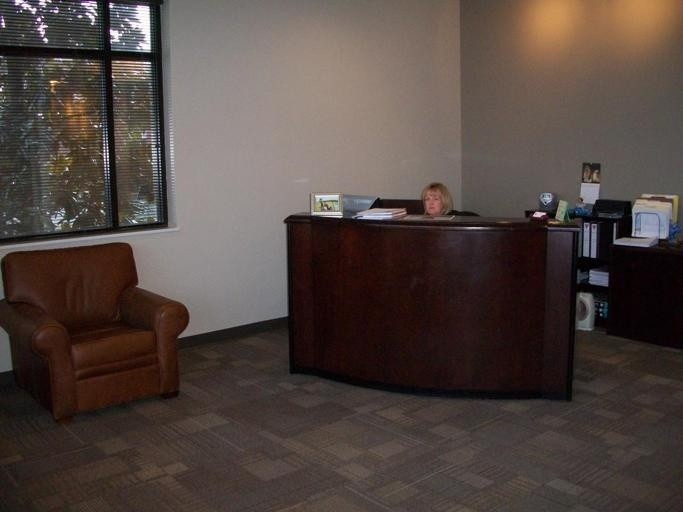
538, 191, 558, 211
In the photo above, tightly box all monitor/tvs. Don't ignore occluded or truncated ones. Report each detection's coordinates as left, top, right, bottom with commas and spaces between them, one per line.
379, 199, 424, 215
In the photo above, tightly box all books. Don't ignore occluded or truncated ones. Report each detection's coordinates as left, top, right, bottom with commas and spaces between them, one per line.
612, 191, 678, 248
587, 267, 608, 287
351, 207, 407, 219
577, 219, 612, 260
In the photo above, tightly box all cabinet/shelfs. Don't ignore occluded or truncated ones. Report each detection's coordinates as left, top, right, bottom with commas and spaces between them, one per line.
525, 198, 683, 352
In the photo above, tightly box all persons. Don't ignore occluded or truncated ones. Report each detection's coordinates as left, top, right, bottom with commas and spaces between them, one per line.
575, 197, 585, 208
420, 181, 454, 217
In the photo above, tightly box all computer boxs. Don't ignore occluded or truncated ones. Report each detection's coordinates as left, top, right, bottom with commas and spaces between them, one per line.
344, 194, 380, 209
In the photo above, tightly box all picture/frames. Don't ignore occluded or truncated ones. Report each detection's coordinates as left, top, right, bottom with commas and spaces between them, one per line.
309, 191, 344, 219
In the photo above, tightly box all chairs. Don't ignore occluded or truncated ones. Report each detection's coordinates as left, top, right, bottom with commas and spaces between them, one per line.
1, 241, 191, 422
